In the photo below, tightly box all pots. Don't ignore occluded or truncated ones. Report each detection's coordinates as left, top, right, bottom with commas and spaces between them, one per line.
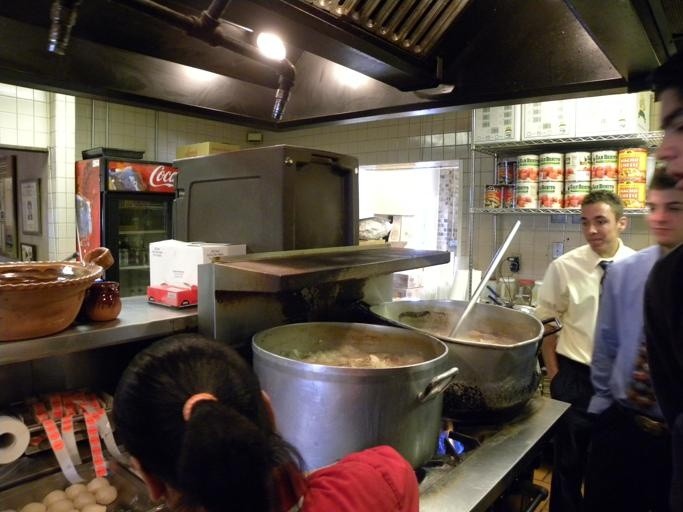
368, 289, 562, 421
250, 323, 450, 473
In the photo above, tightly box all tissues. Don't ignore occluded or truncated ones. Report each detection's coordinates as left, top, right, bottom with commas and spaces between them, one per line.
146, 280, 198, 310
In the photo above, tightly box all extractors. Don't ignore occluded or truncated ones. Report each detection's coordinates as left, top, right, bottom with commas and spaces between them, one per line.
260, 1, 480, 94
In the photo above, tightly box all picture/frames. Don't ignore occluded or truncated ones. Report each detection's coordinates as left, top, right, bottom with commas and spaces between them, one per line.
16, 177, 42, 239
0, 151, 21, 265
16, 242, 37, 264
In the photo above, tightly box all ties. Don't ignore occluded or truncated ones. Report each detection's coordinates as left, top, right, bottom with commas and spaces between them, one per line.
624, 330, 657, 406
597, 260, 615, 300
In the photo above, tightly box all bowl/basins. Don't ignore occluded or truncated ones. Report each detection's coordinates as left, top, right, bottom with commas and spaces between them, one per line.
0, 260, 104, 343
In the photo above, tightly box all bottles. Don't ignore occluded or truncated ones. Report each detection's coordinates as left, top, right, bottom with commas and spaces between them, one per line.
114, 200, 166, 270
497, 277, 543, 307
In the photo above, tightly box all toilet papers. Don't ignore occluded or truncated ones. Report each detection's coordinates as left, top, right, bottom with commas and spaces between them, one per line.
1, 411, 31, 464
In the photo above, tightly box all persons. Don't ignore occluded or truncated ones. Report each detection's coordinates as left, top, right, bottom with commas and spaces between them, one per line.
641, 54, 683, 511
585, 167, 682, 512
533, 190, 638, 511
111, 332, 421, 512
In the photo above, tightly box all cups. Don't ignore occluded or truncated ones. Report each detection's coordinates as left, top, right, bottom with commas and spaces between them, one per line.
84, 280, 123, 324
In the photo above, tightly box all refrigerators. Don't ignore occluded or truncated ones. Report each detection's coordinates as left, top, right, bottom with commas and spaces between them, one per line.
75, 157, 178, 298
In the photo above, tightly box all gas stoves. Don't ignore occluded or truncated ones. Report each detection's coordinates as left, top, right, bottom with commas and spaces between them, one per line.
377, 394, 573, 511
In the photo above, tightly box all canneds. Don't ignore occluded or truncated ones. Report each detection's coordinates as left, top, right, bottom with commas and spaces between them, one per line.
484, 146, 648, 209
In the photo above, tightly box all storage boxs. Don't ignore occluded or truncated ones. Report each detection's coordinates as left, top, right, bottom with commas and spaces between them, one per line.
393, 287, 423, 300
469, 104, 522, 146
145, 239, 247, 291
569, 91, 656, 138
393, 270, 424, 291
176, 138, 241, 163
522, 98, 577, 141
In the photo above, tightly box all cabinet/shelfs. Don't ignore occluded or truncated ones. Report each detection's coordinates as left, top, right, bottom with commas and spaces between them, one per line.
461, 110, 666, 326
